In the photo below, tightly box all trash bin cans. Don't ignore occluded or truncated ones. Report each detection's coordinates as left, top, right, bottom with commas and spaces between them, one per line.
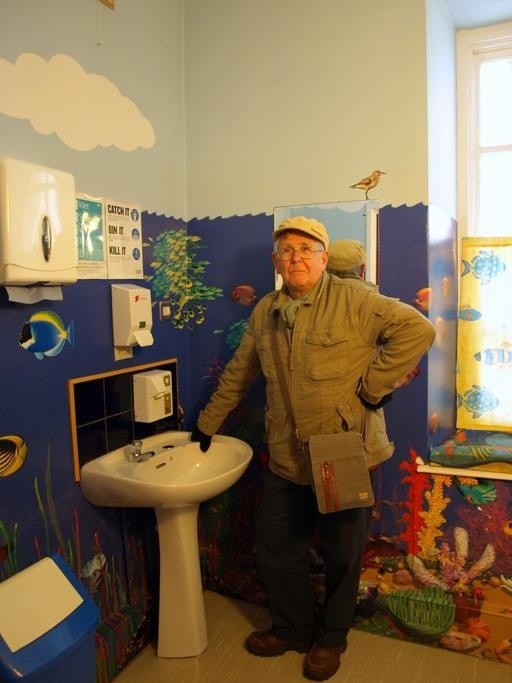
0, 554, 100, 683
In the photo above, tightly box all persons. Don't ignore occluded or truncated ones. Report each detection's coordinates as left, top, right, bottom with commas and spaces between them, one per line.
191, 218, 437, 681
327, 239, 380, 292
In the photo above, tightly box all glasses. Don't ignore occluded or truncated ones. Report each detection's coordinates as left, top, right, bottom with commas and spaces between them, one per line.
273, 244, 326, 262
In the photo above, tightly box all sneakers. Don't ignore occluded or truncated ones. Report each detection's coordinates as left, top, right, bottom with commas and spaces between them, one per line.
304, 638, 347, 681
246, 628, 313, 657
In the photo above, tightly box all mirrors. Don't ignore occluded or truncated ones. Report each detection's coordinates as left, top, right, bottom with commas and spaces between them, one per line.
270, 198, 380, 291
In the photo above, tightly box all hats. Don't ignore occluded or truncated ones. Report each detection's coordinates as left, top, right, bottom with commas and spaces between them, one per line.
271, 216, 329, 251
328, 238, 367, 272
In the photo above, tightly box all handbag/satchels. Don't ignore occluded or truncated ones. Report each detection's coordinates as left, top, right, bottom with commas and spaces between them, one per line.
305, 430, 376, 514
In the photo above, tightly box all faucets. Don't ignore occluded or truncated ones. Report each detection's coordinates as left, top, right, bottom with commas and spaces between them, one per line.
129, 439, 156, 463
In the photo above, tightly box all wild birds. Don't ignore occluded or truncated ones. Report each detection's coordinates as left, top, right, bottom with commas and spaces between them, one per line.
348, 169, 388, 200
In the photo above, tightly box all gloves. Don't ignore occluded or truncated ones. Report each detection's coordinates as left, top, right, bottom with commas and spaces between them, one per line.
190, 425, 212, 453
358, 394, 392, 411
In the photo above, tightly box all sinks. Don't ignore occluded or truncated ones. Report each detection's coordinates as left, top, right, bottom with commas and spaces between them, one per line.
81, 431, 253, 508
